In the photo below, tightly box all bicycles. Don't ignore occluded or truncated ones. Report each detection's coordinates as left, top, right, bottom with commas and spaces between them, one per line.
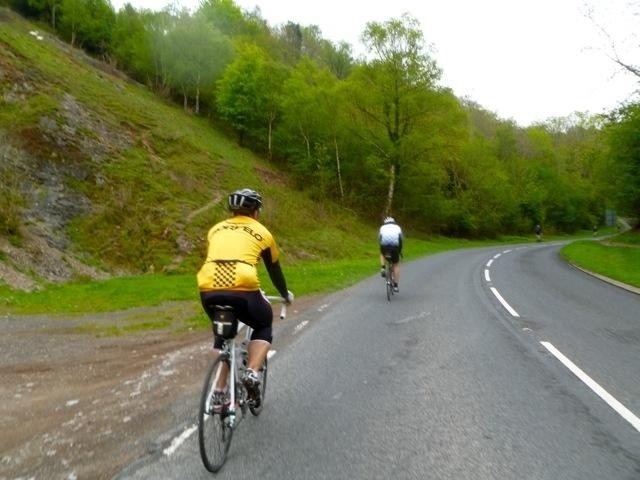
197, 292, 294, 474
383, 252, 404, 300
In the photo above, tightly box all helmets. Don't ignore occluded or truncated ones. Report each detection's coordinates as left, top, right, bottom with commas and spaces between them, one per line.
227, 188, 263, 211
384, 216, 397, 224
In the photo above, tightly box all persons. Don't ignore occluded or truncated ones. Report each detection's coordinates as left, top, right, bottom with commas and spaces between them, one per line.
379, 217, 403, 293
535, 224, 542, 241
196, 188, 295, 409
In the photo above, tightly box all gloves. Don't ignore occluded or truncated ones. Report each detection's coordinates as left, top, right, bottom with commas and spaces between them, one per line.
287, 289, 294, 304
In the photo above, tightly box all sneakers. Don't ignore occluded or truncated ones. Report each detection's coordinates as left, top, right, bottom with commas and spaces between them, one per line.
381, 268, 386, 278
392, 281, 401, 293
239, 367, 263, 409
208, 388, 238, 416
